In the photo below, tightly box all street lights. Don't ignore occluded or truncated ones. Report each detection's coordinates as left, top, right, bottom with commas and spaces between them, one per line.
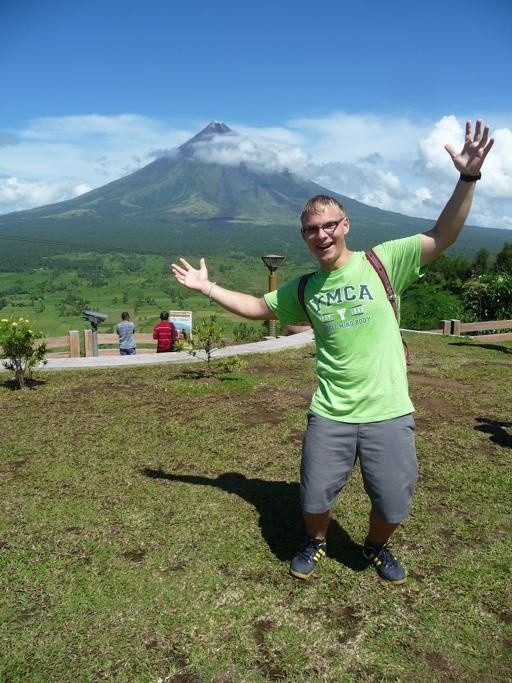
259, 253, 287, 338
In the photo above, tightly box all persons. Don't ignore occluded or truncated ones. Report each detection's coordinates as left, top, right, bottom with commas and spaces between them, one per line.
153, 311, 179, 353
115, 312, 136, 355
170, 118, 495, 585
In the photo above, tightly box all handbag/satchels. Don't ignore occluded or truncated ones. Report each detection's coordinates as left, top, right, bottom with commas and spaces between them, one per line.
171, 340, 178, 352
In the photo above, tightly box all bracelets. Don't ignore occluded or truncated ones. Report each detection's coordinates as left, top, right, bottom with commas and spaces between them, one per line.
460, 172, 482, 182
207, 281, 217, 302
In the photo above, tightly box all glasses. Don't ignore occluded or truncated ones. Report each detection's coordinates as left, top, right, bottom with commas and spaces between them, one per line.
303, 217, 345, 235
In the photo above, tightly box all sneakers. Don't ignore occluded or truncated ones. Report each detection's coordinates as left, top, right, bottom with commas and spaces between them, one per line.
362, 540, 407, 585
289, 537, 327, 578
